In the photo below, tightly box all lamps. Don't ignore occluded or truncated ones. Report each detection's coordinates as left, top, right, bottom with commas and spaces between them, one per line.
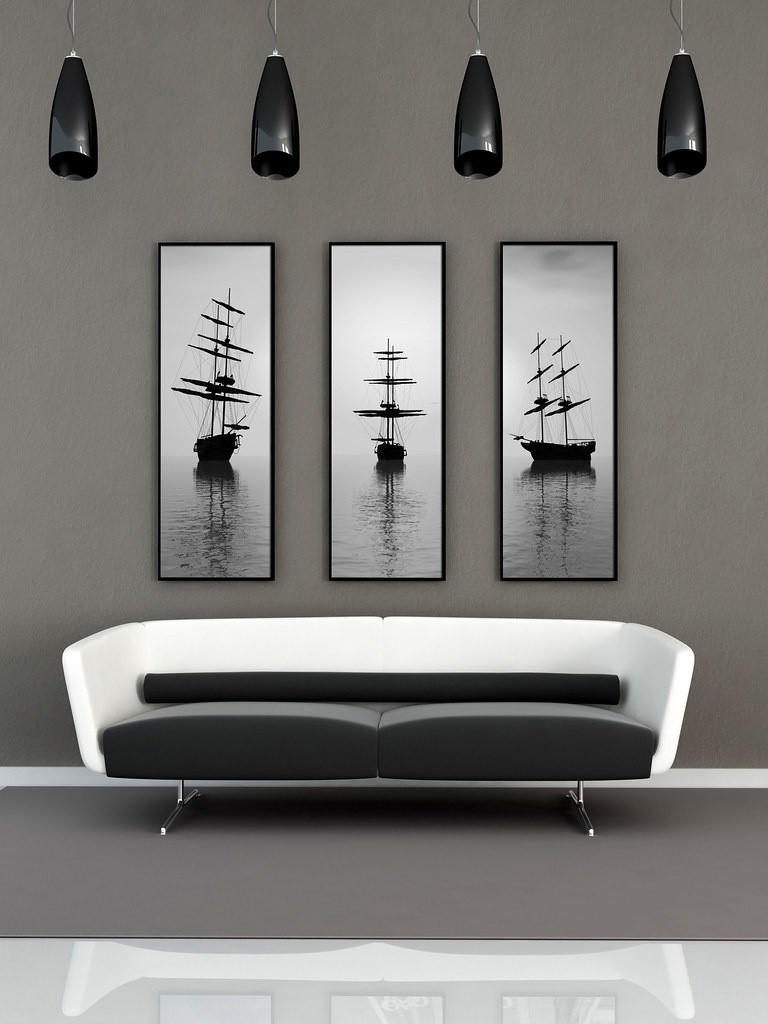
248, 1, 303, 183
47, 0, 99, 183
655, 0, 710, 182
451, 0, 508, 183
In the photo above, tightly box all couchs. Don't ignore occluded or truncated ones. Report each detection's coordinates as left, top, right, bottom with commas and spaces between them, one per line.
59, 614, 697, 838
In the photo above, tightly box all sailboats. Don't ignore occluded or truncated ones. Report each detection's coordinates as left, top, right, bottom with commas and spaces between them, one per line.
353, 337, 428, 470
509, 330, 597, 473
172, 286, 261, 467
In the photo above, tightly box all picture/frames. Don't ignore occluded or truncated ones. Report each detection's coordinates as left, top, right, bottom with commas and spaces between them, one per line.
497, 238, 622, 585
153, 238, 280, 585
327, 240, 450, 583
